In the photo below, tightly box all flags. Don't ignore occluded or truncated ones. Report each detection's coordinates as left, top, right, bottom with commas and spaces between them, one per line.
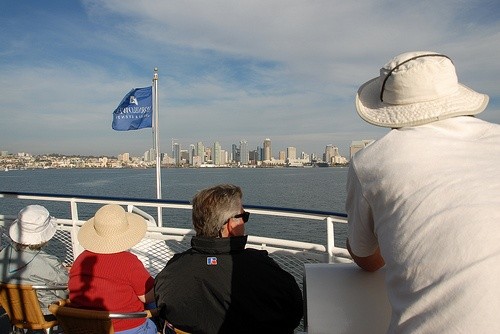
112, 85, 152, 131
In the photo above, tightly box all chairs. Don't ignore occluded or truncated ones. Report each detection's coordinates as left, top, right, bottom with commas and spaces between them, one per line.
48, 298, 152, 334
0, 283, 68, 334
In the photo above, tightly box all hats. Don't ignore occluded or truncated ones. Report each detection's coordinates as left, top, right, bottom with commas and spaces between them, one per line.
355, 51, 490, 127
77, 204, 148, 254
9, 205, 58, 246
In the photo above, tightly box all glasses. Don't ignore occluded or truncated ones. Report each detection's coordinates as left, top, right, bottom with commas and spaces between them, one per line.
223, 212, 250, 225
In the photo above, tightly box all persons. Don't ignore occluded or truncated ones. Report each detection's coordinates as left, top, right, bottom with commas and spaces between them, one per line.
0, 205, 73, 334
154, 183, 304, 334
346, 51, 500, 334
69, 205, 163, 334
